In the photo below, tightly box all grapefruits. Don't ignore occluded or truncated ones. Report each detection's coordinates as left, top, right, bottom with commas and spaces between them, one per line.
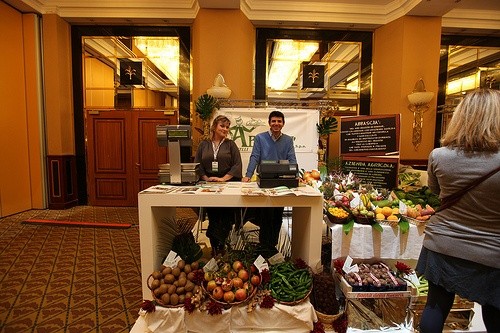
303, 169, 320, 184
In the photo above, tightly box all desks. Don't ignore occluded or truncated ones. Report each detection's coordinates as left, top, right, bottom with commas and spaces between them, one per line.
138, 184, 323, 300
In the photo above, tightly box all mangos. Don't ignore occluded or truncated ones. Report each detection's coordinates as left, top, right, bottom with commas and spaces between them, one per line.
402, 204, 436, 221
371, 199, 415, 207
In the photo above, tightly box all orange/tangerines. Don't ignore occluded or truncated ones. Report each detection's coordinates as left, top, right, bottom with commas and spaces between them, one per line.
375, 206, 399, 221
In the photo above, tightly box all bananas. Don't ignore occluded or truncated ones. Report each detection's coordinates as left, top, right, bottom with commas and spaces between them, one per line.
361, 194, 370, 206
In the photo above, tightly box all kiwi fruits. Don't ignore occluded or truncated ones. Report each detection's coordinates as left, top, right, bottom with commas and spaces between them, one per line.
151, 260, 201, 305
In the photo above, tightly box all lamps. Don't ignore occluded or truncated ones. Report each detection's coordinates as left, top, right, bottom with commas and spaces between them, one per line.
408, 78, 434, 152
207, 73, 231, 98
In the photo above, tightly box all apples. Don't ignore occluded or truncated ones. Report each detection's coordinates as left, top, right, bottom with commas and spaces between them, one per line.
327, 196, 352, 208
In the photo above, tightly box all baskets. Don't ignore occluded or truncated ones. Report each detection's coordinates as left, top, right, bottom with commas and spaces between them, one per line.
201, 274, 262, 304
271, 282, 313, 305
403, 217, 429, 226
375, 218, 401, 226
328, 195, 351, 224
314, 295, 348, 331
147, 274, 185, 307
352, 214, 375, 223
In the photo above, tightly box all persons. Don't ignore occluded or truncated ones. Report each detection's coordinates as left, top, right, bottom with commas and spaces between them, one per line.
195, 116, 242, 257
242, 111, 298, 256
416, 89, 500, 333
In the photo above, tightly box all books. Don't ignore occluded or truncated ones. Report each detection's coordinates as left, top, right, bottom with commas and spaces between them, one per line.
140, 181, 324, 196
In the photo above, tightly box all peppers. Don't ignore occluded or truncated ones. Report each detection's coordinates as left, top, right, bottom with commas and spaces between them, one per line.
416, 274, 429, 294
261, 260, 312, 302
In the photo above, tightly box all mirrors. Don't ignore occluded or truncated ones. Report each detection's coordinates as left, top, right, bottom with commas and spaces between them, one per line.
81, 36, 500, 146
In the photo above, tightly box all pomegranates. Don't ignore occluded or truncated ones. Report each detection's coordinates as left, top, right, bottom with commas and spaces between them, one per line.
205, 261, 259, 302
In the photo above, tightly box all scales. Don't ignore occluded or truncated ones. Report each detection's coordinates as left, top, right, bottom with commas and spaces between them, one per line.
156, 123, 201, 186
